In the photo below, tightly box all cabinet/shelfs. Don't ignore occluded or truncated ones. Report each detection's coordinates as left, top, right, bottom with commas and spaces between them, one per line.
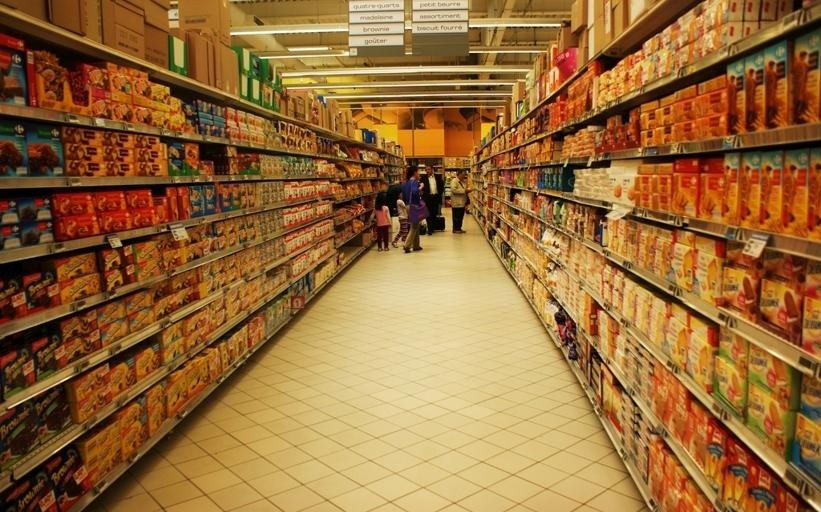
289, 116, 402, 320
172, 73, 287, 427
470, 93, 553, 270
405, 154, 471, 208
2, 6, 172, 510
517, 2, 821, 512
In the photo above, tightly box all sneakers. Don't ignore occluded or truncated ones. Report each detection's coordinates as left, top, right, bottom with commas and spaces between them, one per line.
378, 241, 422, 253
453, 230, 466, 234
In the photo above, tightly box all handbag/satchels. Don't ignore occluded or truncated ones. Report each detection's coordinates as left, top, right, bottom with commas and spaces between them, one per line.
408, 200, 430, 224
465, 193, 470, 205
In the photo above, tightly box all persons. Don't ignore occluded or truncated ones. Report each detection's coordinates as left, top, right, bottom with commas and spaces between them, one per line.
403, 164, 425, 253
368, 193, 392, 254
391, 190, 410, 248
420, 165, 444, 235
451, 167, 472, 233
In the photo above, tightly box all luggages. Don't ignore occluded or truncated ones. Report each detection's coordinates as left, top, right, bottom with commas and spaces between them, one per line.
433, 206, 445, 232
